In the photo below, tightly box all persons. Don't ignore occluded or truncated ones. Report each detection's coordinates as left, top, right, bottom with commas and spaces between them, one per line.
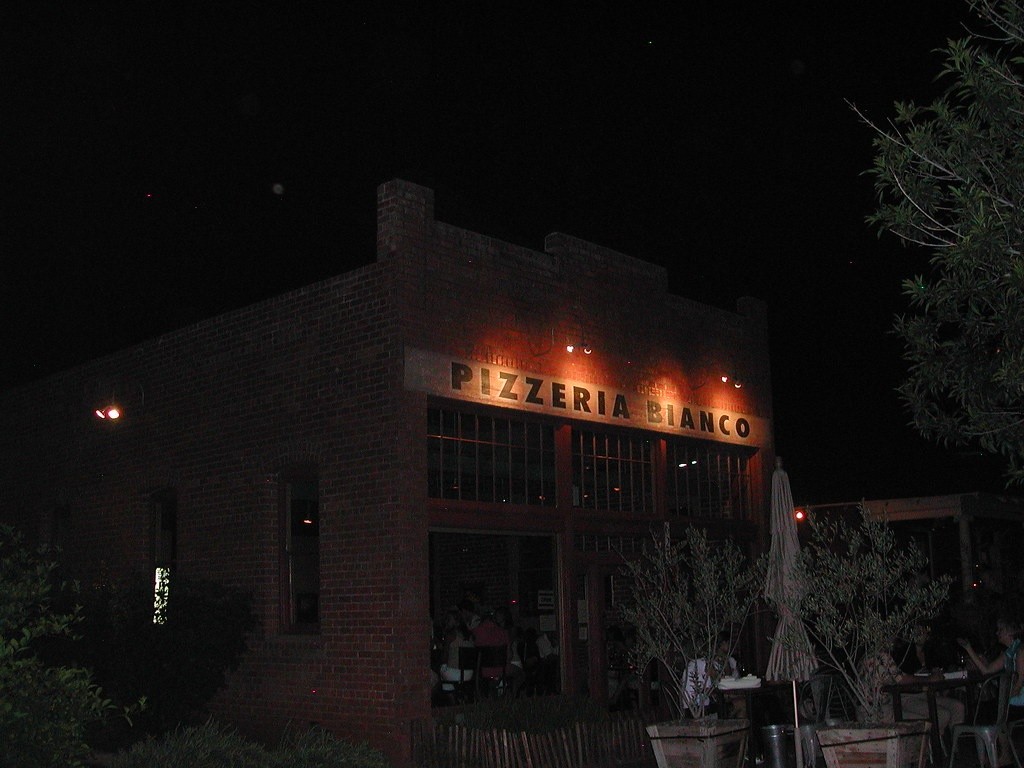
431, 601, 635, 702
679, 613, 1024, 768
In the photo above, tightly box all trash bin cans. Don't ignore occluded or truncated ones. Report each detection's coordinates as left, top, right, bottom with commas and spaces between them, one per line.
800, 722, 829, 768
761, 723, 796, 768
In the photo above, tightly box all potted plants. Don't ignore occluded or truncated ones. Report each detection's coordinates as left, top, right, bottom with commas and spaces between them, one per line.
612, 520, 766, 768
769, 494, 959, 768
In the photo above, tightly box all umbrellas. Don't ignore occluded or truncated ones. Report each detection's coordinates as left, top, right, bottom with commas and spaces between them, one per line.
763, 455, 817, 728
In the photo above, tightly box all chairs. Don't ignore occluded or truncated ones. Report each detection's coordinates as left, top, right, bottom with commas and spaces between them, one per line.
949, 672, 1023, 768
476, 643, 514, 696
440, 643, 483, 703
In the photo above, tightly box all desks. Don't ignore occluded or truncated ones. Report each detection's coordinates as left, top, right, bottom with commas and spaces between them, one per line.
709, 684, 785, 768
881, 674, 1000, 768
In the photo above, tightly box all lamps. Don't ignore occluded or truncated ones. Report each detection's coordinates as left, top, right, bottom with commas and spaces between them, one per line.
93, 386, 122, 421
536, 312, 592, 357
694, 362, 742, 391
